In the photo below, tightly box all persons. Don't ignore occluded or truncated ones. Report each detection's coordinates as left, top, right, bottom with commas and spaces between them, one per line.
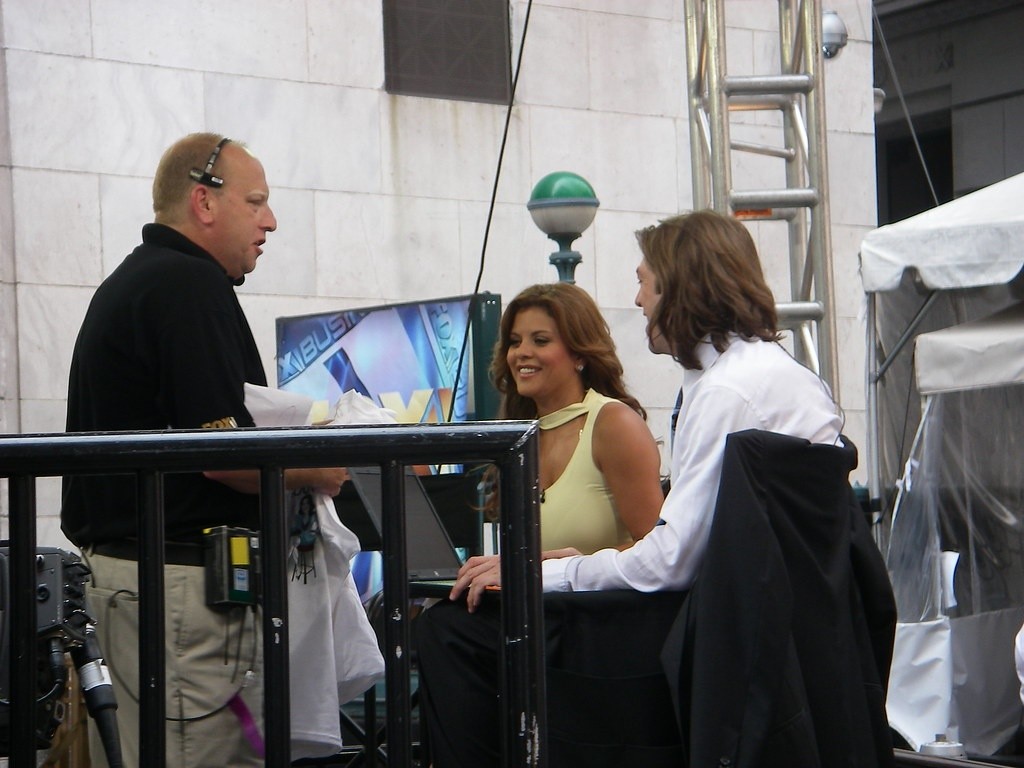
449, 284, 665, 763
447, 210, 897, 768
60, 133, 353, 768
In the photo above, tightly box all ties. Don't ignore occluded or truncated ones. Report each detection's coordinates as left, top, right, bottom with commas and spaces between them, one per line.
671, 387, 683, 457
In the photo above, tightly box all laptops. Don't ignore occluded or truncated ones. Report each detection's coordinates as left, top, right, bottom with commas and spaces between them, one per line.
348, 464, 502, 592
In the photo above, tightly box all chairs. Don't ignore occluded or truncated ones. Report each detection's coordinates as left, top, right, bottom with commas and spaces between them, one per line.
543, 428, 897, 768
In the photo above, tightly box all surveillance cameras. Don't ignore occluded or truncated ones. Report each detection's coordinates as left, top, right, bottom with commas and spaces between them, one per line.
822, 9, 850, 57
